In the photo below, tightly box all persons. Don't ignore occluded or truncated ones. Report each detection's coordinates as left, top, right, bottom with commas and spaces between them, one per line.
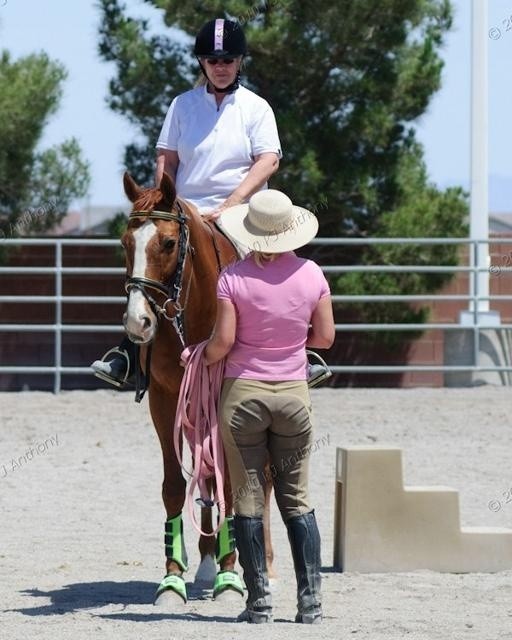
89, 16, 336, 391
176, 186, 341, 626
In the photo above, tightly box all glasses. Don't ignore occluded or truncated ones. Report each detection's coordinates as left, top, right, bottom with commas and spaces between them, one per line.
206, 58, 237, 65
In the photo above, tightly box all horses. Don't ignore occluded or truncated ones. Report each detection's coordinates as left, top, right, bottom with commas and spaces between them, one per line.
116, 168, 278, 609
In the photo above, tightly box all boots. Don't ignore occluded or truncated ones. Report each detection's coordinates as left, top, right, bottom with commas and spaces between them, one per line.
234, 515, 273, 625
284, 509, 324, 625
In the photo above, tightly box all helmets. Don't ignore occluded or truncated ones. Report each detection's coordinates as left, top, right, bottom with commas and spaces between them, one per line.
193, 19, 246, 58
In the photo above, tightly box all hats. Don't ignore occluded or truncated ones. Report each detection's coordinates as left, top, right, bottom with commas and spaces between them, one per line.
220, 189, 320, 253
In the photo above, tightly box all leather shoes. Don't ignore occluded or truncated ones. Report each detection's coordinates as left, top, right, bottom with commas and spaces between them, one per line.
92, 349, 145, 389
310, 364, 324, 382
